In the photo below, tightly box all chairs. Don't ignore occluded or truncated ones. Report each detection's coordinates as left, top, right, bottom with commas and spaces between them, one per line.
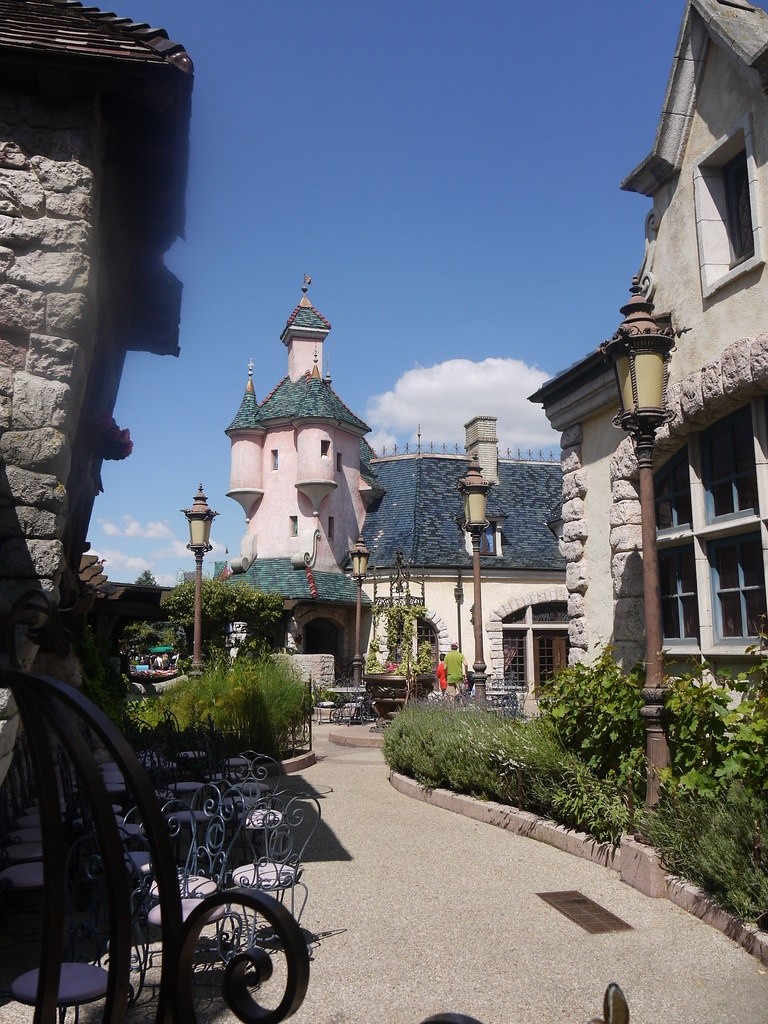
468, 673, 532, 717
316, 676, 372, 725
0, 670, 322, 1024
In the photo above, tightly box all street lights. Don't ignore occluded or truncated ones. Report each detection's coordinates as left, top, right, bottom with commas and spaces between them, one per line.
179, 484, 221, 683
348, 534, 371, 686
459, 449, 494, 714
599, 275, 693, 844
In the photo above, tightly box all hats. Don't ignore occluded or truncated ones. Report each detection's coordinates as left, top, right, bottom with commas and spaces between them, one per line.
450, 643, 458, 648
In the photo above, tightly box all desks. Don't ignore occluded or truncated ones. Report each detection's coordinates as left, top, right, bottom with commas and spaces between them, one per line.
486, 691, 512, 696
327, 686, 368, 695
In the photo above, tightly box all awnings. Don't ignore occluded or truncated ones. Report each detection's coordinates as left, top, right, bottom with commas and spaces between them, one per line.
151, 646, 175, 652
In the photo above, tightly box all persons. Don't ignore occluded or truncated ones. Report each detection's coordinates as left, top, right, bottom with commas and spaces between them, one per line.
444, 642, 468, 696
436, 653, 447, 695
153, 653, 169, 670
